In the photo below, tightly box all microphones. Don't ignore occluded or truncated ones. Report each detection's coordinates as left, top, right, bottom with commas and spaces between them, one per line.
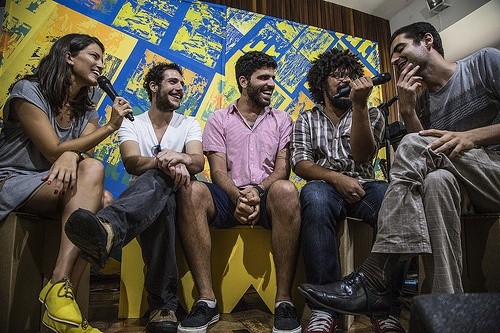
377, 95, 399, 111
333, 72, 391, 100
98, 76, 134, 122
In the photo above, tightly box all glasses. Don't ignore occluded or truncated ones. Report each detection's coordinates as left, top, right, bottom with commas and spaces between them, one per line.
329, 72, 359, 79
151, 145, 161, 157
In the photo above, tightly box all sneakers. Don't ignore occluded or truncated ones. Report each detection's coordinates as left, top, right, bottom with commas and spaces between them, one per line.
303, 308, 337, 333
65, 209, 114, 267
370, 315, 406, 333
147, 309, 177, 333
177, 300, 220, 333
42, 309, 102, 333
38, 278, 83, 326
272, 302, 303, 333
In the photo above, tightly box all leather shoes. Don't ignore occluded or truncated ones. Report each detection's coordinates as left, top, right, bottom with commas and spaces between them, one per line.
297, 268, 391, 320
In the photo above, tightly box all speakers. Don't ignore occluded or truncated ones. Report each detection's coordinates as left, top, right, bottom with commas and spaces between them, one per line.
425, 0, 450, 14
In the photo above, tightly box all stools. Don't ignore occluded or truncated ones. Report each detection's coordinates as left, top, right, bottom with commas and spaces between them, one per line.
0, 211, 499, 333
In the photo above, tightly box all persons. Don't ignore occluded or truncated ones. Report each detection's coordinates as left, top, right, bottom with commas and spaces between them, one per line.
0, 33, 133, 333
64, 62, 205, 333
298, 22, 500, 321
178, 51, 302, 333
290, 48, 407, 333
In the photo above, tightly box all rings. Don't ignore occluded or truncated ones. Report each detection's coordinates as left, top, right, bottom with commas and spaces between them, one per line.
352, 192, 358, 196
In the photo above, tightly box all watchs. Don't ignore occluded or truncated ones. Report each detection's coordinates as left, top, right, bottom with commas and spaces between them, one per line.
253, 184, 266, 200
71, 150, 84, 163
